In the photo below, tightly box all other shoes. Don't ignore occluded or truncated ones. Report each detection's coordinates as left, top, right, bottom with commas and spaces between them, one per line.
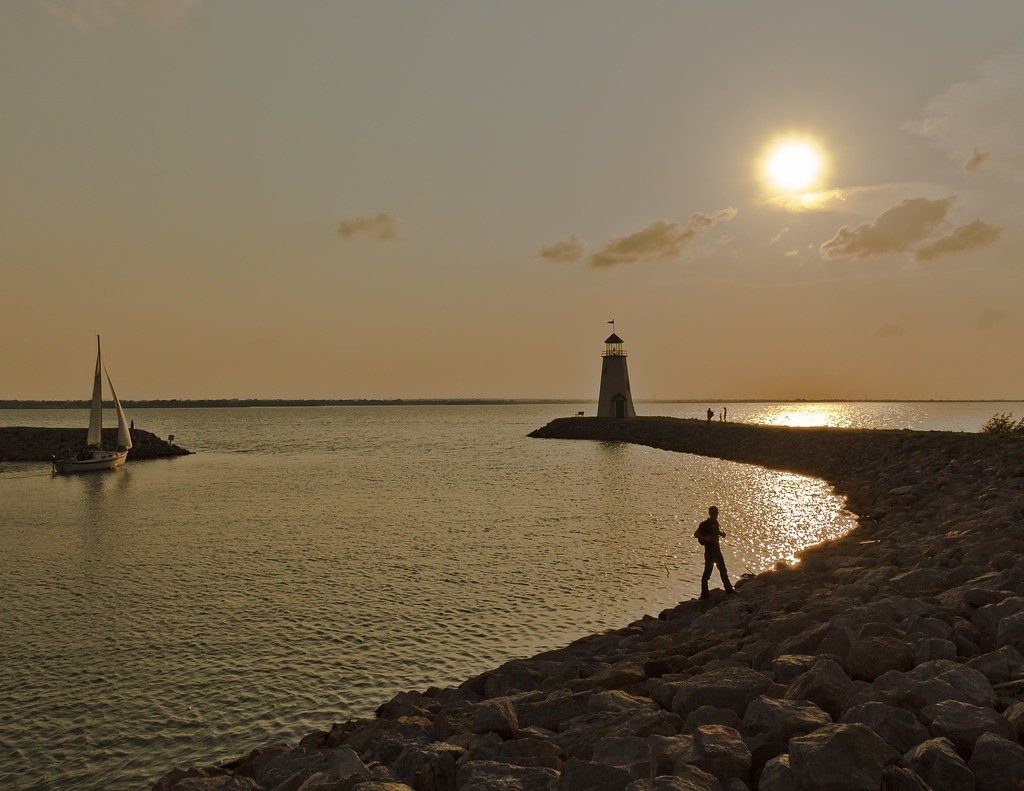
701, 592, 709, 601
725, 587, 737, 595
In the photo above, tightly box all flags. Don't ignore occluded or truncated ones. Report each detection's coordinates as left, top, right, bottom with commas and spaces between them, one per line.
606, 321, 613, 323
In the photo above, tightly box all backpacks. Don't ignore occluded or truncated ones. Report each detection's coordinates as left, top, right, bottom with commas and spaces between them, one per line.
697, 518, 725, 546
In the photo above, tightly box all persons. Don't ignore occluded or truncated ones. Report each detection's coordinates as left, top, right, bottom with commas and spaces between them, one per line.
694, 506, 740, 602
707, 408, 714, 423
724, 407, 726, 422
720, 413, 722, 422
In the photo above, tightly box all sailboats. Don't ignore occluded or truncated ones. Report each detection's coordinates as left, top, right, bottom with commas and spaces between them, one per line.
52, 335, 132, 478
